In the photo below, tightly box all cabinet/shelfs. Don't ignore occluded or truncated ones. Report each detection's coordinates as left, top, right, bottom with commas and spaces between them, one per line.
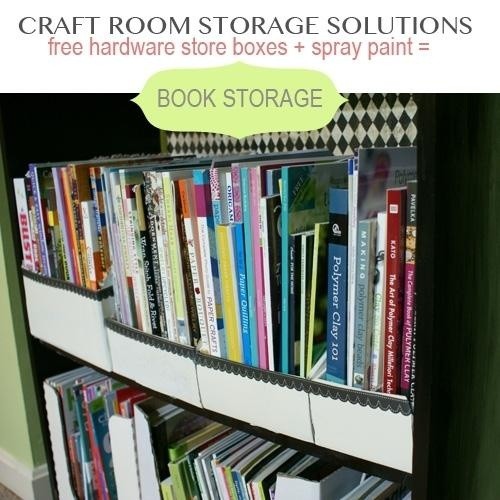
21, 93, 499, 500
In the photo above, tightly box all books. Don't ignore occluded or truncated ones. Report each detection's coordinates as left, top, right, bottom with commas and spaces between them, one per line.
44, 366, 214, 499
100, 146, 418, 398
161, 421, 412, 499
13, 154, 196, 290
